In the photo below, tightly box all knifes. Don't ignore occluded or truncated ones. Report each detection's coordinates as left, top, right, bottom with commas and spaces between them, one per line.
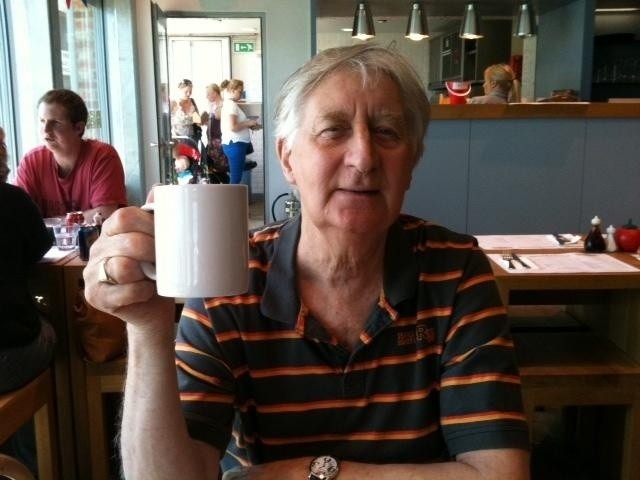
553, 232, 567, 246
511, 252, 532, 270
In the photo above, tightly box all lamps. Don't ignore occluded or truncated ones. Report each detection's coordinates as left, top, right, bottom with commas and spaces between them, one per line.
516, 0, 537, 38
458, 0, 487, 40
404, 0, 431, 42
351, 0, 376, 41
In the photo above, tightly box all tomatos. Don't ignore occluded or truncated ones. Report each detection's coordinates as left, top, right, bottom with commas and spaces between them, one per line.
615, 223, 640, 252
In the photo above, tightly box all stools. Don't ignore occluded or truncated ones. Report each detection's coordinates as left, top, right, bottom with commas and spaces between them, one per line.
506, 329, 640, 480
0, 365, 57, 480
83, 363, 126, 480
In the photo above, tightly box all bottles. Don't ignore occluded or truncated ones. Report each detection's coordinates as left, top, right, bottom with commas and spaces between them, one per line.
614, 218, 640, 253
77, 224, 100, 261
604, 225, 618, 254
283, 192, 301, 220
92, 212, 104, 229
583, 214, 607, 254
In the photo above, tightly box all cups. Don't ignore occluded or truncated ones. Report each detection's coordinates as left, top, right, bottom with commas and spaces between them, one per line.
42, 217, 63, 246
134, 182, 252, 299
51, 222, 81, 251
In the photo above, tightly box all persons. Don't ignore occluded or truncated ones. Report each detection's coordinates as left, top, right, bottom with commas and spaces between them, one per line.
81, 45, 531, 480
16, 89, 127, 224
1, 129, 60, 479
161, 78, 257, 220
471, 62, 520, 103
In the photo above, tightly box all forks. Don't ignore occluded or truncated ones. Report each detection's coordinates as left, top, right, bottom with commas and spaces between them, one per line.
502, 248, 516, 271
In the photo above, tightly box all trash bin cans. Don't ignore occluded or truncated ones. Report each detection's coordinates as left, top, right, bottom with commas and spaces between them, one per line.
227, 158, 257, 205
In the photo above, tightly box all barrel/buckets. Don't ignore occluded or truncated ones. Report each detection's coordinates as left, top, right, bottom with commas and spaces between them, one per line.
444, 81, 472, 105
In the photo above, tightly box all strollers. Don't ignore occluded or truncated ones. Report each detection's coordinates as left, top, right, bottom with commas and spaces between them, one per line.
172, 126, 210, 185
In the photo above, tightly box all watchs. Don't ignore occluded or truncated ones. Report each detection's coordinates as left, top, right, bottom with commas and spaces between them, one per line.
310, 453, 340, 479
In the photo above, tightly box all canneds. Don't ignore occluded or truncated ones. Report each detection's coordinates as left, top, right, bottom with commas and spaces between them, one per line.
66, 211, 84, 244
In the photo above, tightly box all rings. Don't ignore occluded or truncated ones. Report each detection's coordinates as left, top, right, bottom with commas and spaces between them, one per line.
98, 257, 116, 286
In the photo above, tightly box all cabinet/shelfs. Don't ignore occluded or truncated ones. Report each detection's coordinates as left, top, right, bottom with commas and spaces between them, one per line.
428, 35, 442, 89
442, 31, 463, 88
463, 19, 513, 83
